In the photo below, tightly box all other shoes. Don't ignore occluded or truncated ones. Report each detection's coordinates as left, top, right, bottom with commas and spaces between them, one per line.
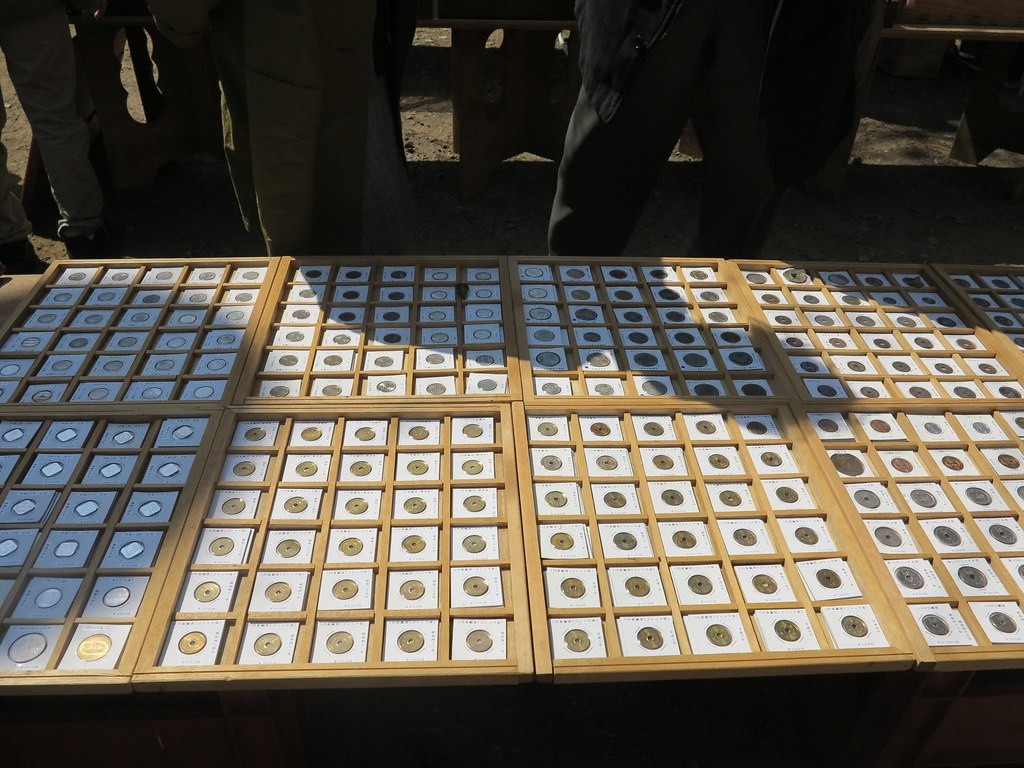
0, 237, 50, 273
65, 222, 113, 259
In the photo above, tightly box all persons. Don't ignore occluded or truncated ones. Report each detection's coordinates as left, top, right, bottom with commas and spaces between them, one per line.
548, 0, 879, 258
0, 0, 107, 274
145, 1, 431, 256
